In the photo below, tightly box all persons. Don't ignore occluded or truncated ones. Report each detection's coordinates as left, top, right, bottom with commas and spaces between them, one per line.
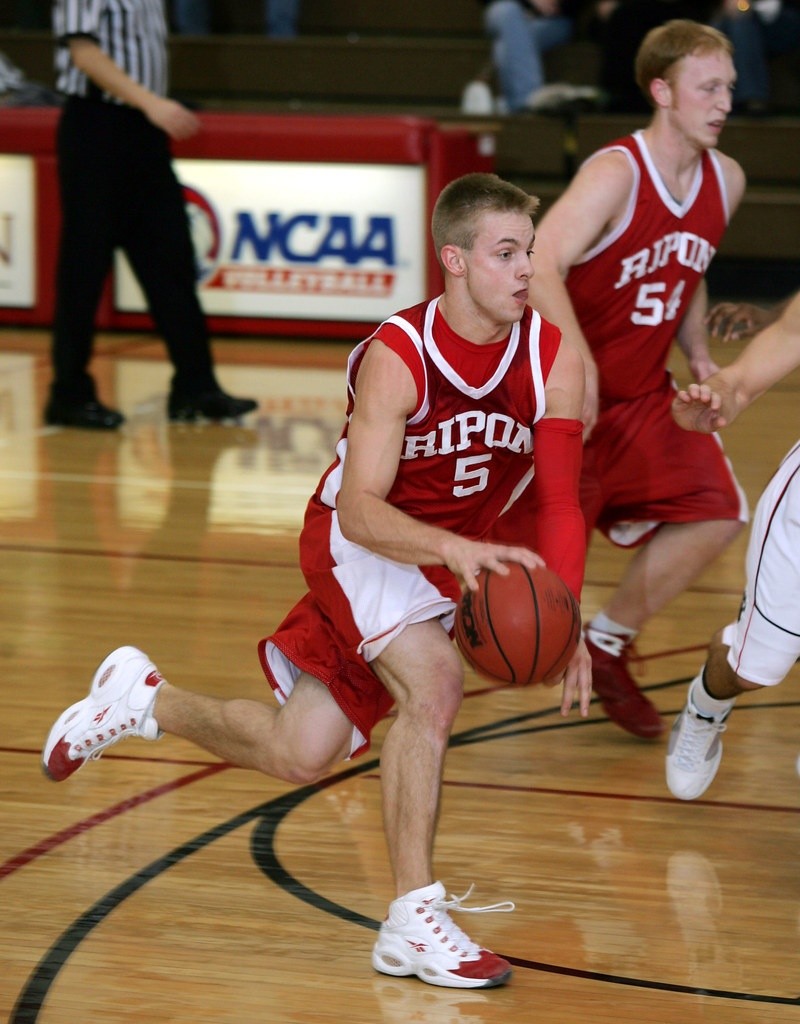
43, 0, 261, 430
175, 0, 800, 113
661, 292, 800, 802
41, 174, 581, 991
526, 21, 754, 740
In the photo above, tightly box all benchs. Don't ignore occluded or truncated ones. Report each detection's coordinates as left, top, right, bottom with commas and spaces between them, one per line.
0, 0, 800, 305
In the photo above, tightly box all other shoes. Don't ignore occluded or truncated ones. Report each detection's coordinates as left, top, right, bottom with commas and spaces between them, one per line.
167, 387, 255, 421
40, 395, 125, 431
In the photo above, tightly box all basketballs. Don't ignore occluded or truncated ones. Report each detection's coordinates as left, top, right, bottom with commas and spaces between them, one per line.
454, 558, 584, 687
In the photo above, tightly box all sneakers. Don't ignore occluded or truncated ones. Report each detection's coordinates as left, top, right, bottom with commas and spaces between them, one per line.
39, 644, 168, 784
665, 676, 735, 802
582, 621, 662, 739
369, 882, 518, 989
